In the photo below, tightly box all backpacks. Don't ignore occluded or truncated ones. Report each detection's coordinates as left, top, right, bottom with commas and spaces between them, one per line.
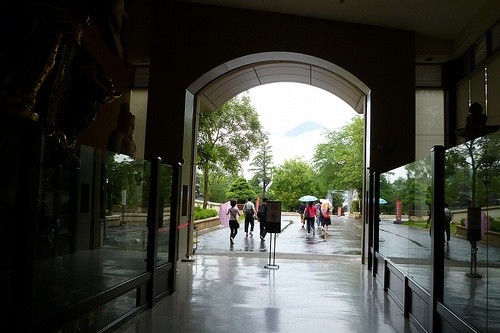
257, 203, 266, 219
324, 211, 329, 218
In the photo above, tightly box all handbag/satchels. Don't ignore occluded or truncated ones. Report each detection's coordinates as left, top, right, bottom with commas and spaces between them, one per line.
246, 208, 254, 217
304, 211, 309, 220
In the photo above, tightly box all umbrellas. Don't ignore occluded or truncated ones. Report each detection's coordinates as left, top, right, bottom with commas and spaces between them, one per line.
321, 200, 328, 214
298, 195, 318, 202
219, 201, 238, 226
380, 198, 387, 204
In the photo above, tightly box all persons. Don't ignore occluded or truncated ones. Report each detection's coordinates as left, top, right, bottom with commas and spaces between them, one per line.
300, 201, 332, 240
227, 200, 240, 249
257, 198, 268, 241
243, 197, 257, 238
426, 203, 452, 245
